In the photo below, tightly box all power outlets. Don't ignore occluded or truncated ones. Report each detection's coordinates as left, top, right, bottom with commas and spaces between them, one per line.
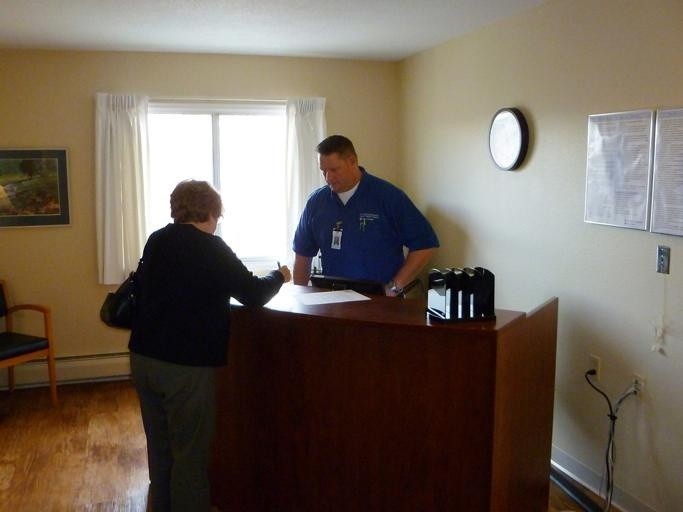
588, 354, 601, 381
632, 374, 646, 400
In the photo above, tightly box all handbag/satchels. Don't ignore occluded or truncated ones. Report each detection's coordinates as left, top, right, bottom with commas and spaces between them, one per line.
100, 271, 139, 332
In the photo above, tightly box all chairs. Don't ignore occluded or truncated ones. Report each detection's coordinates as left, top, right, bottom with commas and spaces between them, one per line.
0, 279, 58, 408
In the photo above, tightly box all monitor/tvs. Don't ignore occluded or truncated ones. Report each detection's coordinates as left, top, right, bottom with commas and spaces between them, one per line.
310, 275, 386, 297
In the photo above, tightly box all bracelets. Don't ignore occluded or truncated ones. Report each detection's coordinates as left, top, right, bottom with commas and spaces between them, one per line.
385, 278, 404, 297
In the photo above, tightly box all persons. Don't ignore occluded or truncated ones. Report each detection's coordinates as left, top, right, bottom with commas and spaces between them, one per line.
121, 175, 292, 511
291, 131, 441, 301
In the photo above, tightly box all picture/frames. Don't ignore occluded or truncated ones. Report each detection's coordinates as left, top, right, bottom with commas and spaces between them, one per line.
0, 148, 72, 229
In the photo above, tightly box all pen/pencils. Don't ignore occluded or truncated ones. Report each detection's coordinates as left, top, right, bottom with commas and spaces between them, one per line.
277, 262, 281, 269
